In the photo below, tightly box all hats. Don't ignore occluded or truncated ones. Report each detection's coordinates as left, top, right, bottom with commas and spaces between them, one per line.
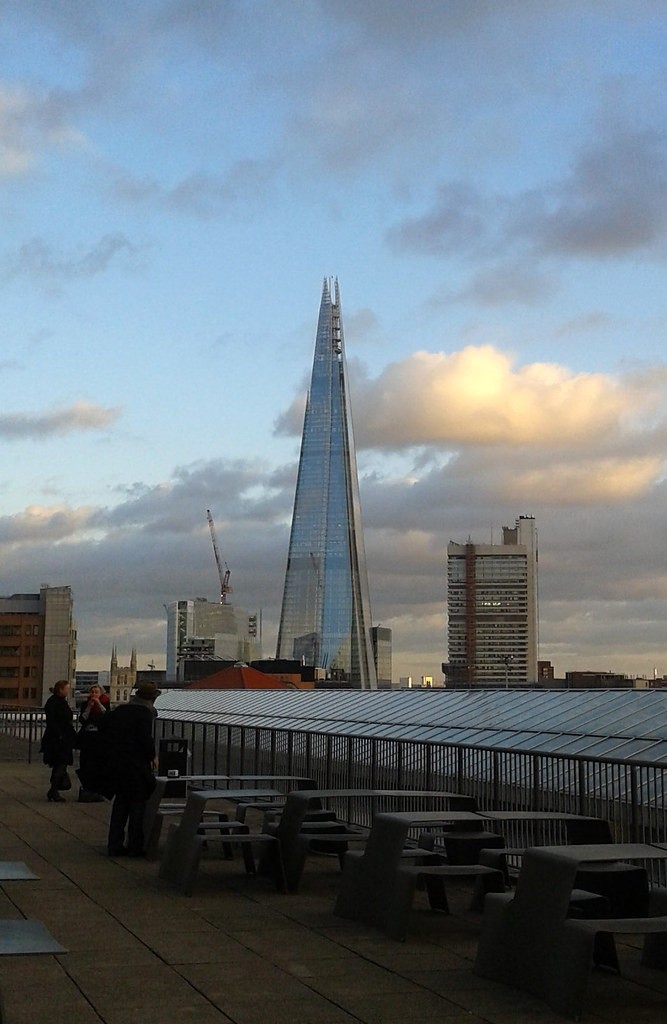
134, 679, 161, 700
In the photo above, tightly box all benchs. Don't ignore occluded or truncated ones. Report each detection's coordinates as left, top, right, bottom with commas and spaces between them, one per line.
138, 774, 667, 1002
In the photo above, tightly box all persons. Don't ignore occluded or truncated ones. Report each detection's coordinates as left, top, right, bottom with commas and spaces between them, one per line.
107, 681, 160, 857
39, 680, 77, 802
74, 686, 111, 802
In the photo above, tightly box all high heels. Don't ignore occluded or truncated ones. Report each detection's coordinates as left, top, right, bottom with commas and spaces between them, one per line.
46, 788, 66, 803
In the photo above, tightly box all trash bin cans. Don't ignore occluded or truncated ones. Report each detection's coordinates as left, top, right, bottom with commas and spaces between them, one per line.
156, 735, 189, 798
